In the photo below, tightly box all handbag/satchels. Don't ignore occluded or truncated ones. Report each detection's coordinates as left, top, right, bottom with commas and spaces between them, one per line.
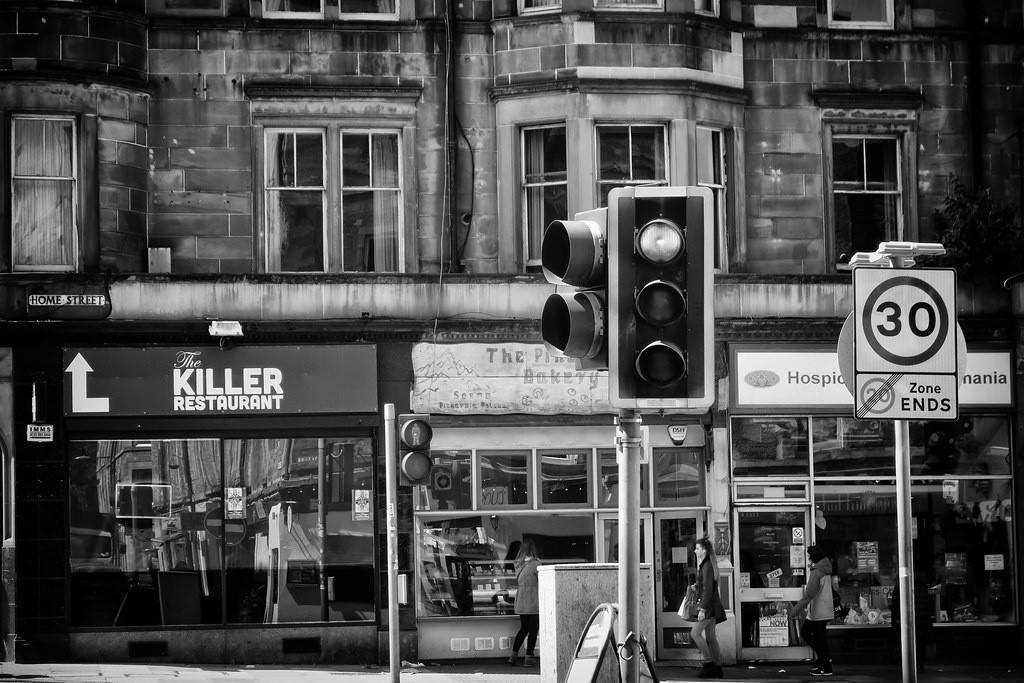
677, 586, 700, 623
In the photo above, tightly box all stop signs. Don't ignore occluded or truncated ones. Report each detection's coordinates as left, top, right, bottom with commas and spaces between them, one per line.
204, 508, 246, 545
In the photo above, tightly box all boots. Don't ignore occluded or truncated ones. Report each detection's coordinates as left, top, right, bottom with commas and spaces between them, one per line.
507, 650, 520, 665
524, 654, 539, 668
697, 662, 723, 678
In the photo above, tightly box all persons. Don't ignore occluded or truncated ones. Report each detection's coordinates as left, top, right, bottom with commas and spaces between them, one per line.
740, 551, 768, 648
503, 540, 525, 615
507, 539, 543, 667
891, 565, 930, 675
663, 529, 686, 608
689, 534, 727, 678
789, 544, 835, 675
613, 544, 618, 561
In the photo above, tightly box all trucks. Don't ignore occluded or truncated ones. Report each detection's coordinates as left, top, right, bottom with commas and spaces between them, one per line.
66, 513, 126, 576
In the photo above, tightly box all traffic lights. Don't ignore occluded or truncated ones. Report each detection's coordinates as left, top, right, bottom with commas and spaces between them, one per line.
540, 208, 613, 374
398, 412, 433, 486
610, 184, 715, 408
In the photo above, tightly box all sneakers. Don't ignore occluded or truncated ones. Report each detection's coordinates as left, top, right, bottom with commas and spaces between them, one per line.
809, 657, 834, 675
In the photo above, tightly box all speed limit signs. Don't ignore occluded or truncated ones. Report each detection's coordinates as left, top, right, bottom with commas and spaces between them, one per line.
851, 265, 959, 420
